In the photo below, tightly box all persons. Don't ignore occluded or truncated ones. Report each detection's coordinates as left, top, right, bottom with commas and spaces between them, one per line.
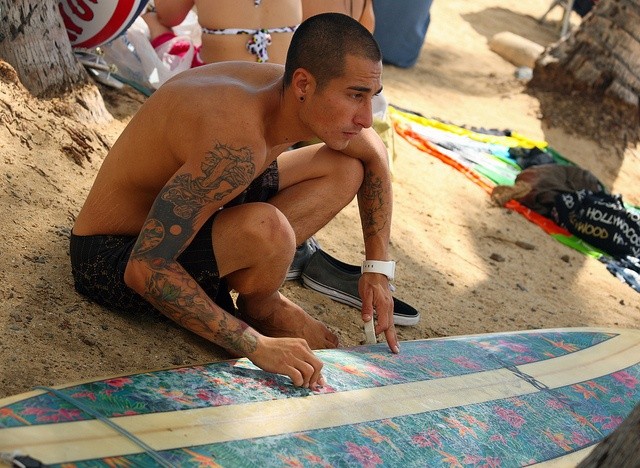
303, 1, 375, 35
70, 13, 401, 388
141, 0, 304, 72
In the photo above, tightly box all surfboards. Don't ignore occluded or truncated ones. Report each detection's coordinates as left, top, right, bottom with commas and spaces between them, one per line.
0, 326, 639, 468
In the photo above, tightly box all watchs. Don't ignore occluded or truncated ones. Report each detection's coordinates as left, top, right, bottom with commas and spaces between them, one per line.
361, 259, 397, 282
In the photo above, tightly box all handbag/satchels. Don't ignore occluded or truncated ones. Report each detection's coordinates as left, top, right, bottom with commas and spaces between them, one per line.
552, 188, 640, 264
507, 147, 557, 169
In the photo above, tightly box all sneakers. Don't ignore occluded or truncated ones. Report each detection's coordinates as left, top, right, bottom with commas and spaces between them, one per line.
284, 238, 322, 281
301, 249, 420, 327
